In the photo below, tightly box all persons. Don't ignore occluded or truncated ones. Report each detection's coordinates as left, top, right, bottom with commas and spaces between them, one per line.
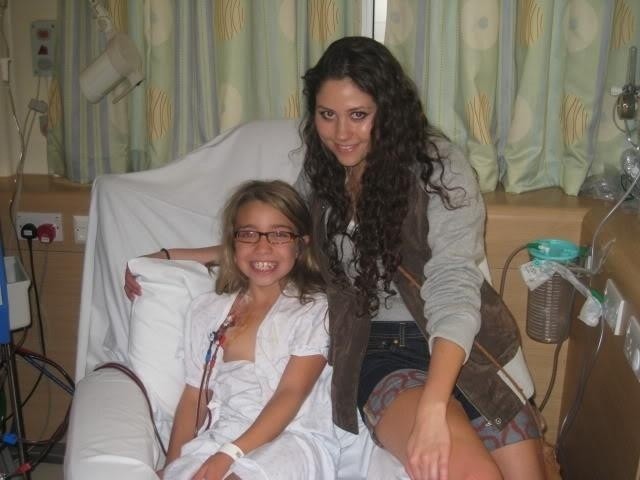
156, 178, 343, 480
124, 34, 547, 480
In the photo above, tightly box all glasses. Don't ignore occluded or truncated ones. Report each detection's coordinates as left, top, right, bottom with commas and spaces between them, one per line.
233, 230, 300, 245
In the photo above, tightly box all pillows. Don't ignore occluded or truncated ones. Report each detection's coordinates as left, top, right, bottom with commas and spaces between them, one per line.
123, 254, 224, 420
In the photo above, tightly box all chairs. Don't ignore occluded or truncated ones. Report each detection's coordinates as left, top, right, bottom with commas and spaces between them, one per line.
64, 120, 413, 480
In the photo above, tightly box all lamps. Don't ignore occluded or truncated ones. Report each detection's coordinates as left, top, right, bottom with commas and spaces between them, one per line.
75, 0, 146, 106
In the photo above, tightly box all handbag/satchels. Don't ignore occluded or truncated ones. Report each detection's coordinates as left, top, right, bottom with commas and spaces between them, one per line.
540, 437, 564, 480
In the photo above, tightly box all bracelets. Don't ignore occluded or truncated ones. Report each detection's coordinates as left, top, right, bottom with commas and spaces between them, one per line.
160, 247, 171, 261
215, 443, 245, 463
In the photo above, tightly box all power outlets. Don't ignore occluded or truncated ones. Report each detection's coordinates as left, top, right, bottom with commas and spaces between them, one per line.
16, 211, 64, 243
